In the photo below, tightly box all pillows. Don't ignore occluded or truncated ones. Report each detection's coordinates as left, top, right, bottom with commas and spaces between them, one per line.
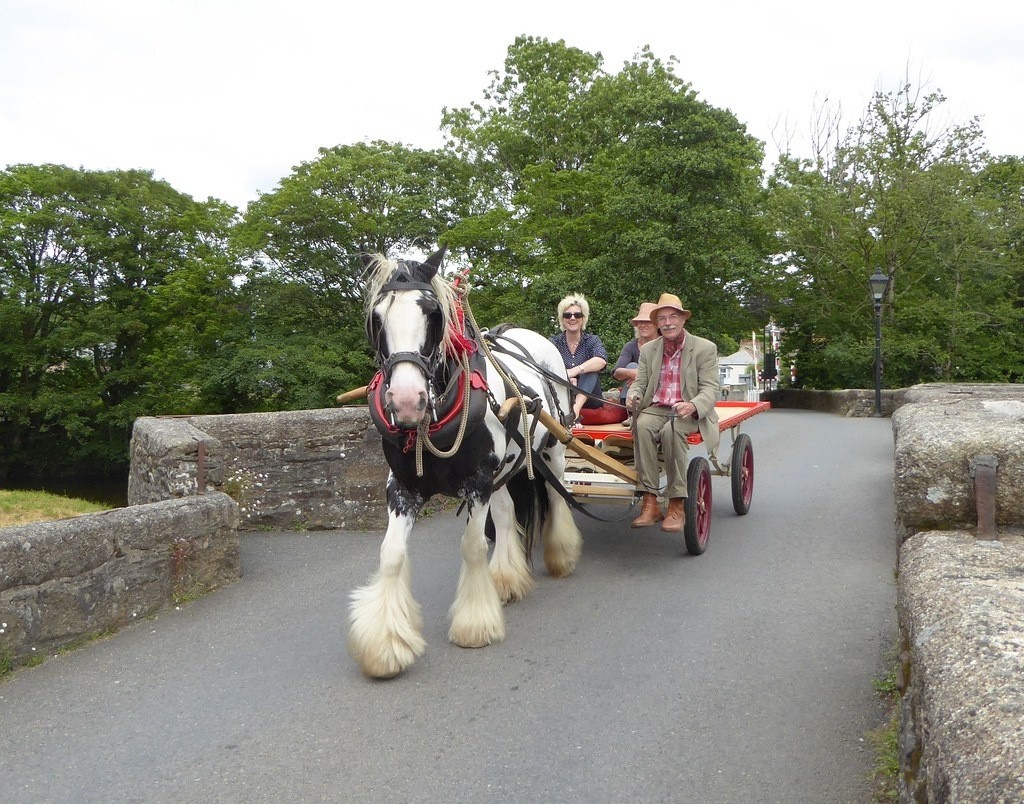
578, 400, 627, 425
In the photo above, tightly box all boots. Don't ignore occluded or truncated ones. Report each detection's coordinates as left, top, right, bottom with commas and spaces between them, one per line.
662, 499, 685, 532
631, 494, 665, 528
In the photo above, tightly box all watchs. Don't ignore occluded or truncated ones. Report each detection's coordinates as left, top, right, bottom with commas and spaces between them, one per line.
578, 365, 584, 374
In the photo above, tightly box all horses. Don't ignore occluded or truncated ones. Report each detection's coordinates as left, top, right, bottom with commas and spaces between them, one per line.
344, 241, 586, 683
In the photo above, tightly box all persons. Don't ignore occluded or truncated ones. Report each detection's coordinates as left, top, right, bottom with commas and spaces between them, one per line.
610, 302, 662, 426
545, 292, 610, 422
624, 292, 721, 532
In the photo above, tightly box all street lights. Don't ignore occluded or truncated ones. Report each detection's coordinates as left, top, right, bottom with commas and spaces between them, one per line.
865, 266, 889, 418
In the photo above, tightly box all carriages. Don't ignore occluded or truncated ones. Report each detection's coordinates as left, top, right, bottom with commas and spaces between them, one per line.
337, 233, 772, 680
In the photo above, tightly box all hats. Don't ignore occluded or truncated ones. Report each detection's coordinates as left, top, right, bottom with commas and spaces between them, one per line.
631, 303, 658, 325
649, 294, 690, 326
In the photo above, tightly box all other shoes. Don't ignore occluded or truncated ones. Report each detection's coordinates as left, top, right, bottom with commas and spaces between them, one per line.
622, 415, 632, 425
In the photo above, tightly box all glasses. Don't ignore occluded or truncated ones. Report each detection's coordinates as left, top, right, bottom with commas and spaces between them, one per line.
563, 312, 584, 318
